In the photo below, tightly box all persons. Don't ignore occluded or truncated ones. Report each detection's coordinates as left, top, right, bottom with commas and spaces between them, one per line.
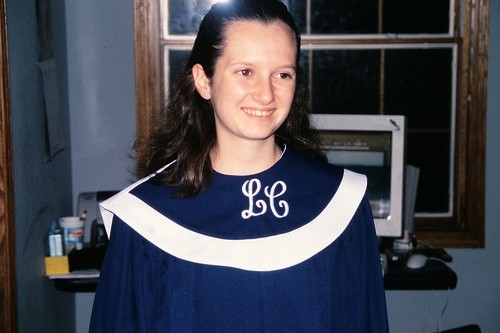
88, 0, 390, 333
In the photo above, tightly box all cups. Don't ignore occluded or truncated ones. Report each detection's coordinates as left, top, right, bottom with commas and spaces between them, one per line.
58, 216, 85, 247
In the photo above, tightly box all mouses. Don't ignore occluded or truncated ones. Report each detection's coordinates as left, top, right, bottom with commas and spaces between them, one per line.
406, 253, 429, 270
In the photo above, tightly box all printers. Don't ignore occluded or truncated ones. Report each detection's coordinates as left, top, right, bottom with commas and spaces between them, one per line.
75, 191, 125, 241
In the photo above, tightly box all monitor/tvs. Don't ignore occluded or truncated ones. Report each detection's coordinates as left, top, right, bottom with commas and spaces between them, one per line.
300, 113, 406, 238
